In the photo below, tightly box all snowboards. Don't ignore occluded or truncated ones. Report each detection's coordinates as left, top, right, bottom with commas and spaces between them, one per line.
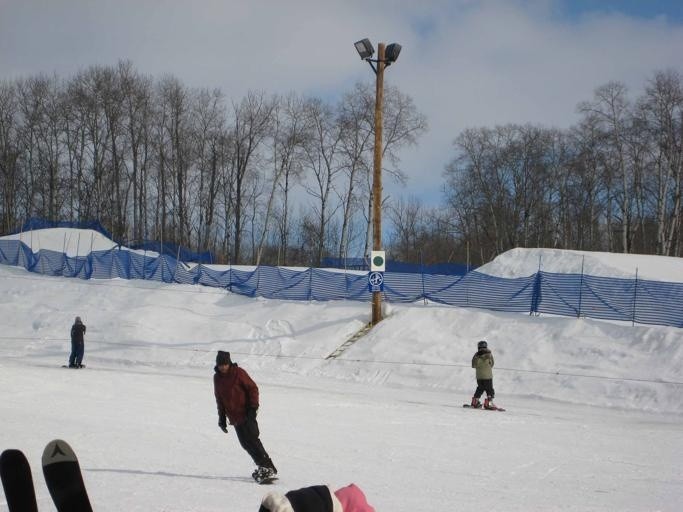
252, 474, 279, 484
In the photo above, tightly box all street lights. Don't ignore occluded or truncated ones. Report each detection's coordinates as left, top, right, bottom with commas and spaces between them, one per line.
354, 38, 401, 325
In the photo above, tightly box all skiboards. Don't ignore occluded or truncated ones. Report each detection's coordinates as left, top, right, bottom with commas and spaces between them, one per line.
464, 404, 506, 411
1, 439, 93, 512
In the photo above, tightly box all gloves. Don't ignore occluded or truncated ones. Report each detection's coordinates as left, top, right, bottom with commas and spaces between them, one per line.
246, 406, 258, 420
218, 421, 228, 433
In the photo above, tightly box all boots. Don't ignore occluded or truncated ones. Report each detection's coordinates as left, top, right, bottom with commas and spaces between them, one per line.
471, 397, 498, 410
252, 465, 278, 483
68, 362, 84, 368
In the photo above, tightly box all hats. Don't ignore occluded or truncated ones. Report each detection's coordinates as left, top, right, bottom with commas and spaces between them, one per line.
74, 317, 82, 324
478, 340, 488, 350
216, 350, 233, 368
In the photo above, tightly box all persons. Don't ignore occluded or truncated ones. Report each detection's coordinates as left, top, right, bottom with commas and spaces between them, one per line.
213, 350, 277, 482
68, 317, 86, 368
471, 341, 498, 410
